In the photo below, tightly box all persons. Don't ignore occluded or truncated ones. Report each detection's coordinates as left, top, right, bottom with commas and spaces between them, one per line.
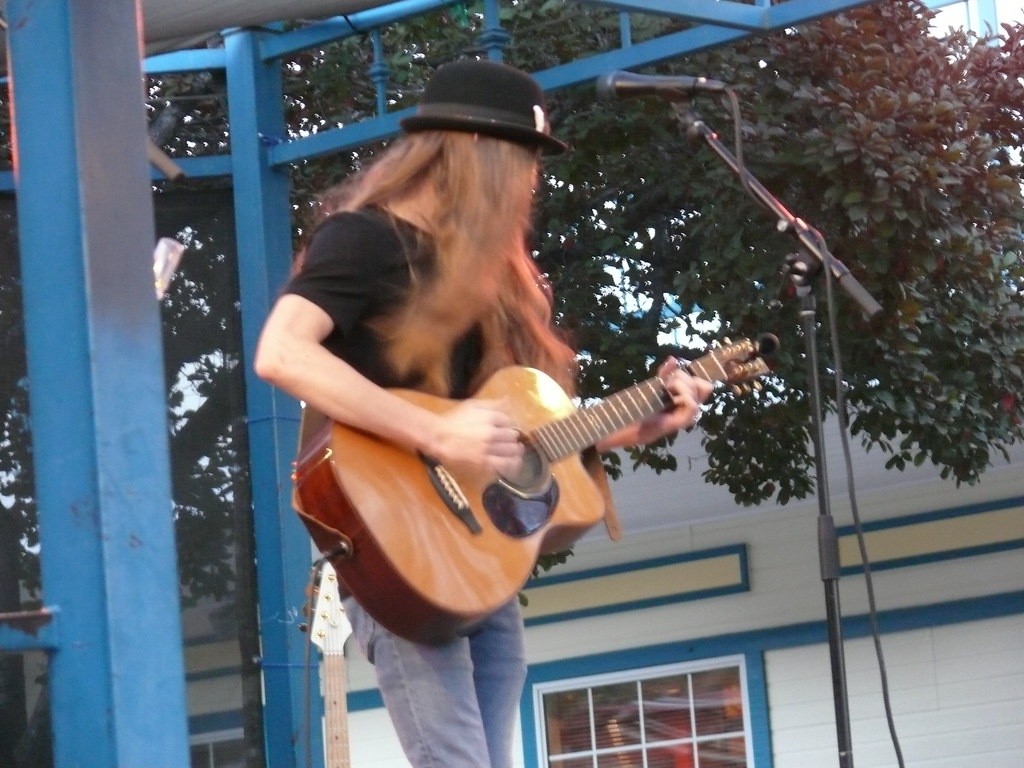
253, 59, 714, 768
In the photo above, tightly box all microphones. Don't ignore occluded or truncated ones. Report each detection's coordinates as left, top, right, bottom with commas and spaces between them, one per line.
594, 66, 727, 97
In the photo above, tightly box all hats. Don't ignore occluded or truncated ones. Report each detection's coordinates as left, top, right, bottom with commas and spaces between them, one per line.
401, 60, 566, 155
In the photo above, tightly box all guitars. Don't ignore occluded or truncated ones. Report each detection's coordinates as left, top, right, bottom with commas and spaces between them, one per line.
299, 557, 355, 766
296, 333, 772, 648
